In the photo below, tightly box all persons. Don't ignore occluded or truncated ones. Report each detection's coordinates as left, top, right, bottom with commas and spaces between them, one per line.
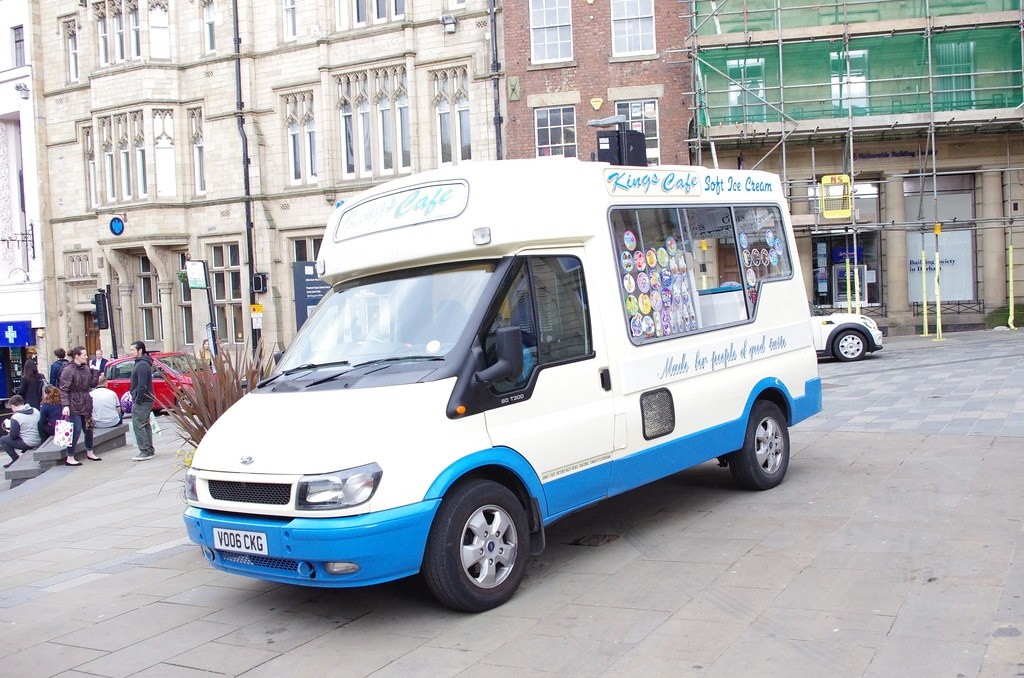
60, 346, 102, 465
129, 342, 155, 460
50, 348, 70, 387
18, 359, 45, 411
38, 386, 63, 443
89, 376, 123, 428
0, 394, 41, 468
92, 350, 108, 373
197, 339, 211, 366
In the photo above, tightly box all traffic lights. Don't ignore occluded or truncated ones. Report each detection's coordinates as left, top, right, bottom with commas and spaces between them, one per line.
91, 293, 108, 329
252, 274, 263, 293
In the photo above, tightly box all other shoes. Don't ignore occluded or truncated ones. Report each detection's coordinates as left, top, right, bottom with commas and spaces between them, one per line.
65, 461, 82, 466
87, 456, 102, 461
3, 459, 17, 468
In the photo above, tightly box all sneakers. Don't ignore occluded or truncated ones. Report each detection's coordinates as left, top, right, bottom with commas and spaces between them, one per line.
133, 450, 155, 460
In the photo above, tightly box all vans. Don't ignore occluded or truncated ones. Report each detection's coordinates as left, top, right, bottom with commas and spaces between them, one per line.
182, 161, 822, 611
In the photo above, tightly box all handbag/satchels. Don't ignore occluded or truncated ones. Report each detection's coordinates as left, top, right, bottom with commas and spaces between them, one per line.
54, 414, 74, 447
128, 411, 160, 448
39, 374, 50, 400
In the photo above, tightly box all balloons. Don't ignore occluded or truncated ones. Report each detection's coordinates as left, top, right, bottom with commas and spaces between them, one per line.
120, 391, 134, 413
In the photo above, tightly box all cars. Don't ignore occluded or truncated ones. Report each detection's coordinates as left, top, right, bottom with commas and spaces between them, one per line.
103, 353, 221, 414
807, 302, 884, 361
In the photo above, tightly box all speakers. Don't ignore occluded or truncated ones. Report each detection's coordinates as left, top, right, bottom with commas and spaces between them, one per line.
626, 130, 649, 168
597, 130, 621, 165
